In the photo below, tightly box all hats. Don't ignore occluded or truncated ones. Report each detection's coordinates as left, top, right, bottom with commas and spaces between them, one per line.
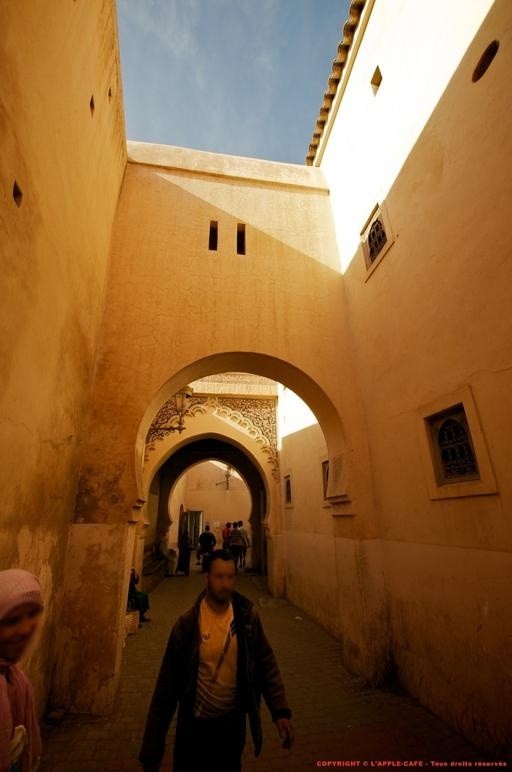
0, 569, 43, 618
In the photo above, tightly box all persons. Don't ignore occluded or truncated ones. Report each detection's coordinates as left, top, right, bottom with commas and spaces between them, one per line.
0, 569, 44, 772
139, 549, 295, 772
128, 568, 151, 622
175, 521, 250, 576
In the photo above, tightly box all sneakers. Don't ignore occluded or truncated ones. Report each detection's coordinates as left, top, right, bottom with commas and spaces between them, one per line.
139, 616, 150, 622
